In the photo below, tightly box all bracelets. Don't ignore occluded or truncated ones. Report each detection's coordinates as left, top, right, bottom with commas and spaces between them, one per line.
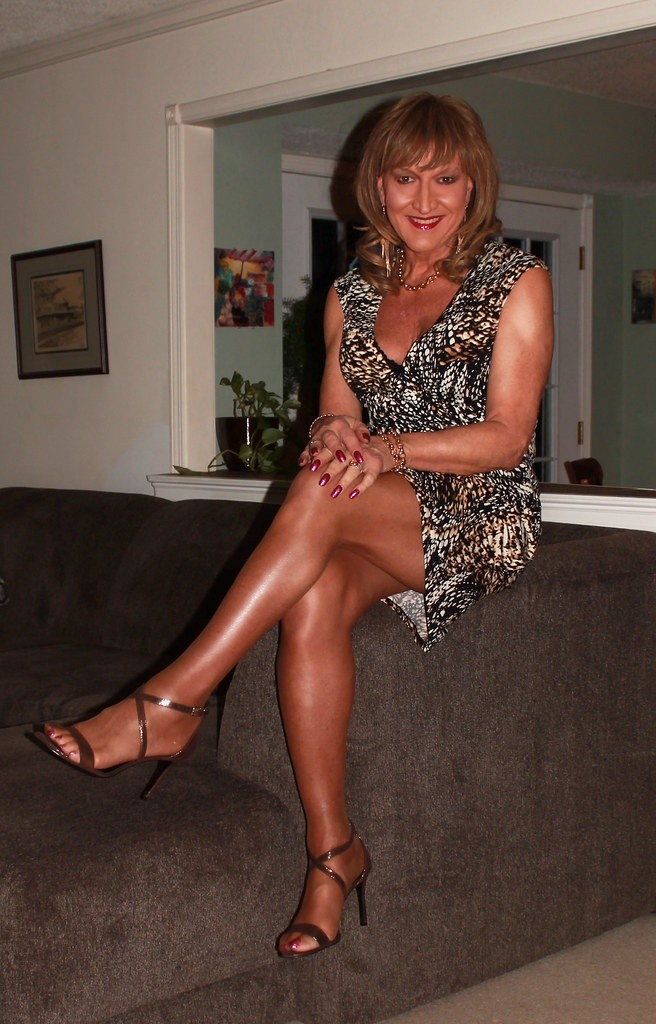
376, 428, 406, 473
309, 413, 336, 439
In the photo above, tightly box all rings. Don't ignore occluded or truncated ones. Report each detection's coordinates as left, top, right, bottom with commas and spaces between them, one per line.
350, 459, 363, 475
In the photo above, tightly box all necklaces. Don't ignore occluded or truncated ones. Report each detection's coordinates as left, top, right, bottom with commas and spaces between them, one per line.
398, 243, 462, 291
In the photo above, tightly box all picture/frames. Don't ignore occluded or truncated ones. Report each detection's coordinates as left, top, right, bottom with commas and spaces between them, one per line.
213, 248, 276, 329
10, 238, 110, 381
630, 268, 655, 326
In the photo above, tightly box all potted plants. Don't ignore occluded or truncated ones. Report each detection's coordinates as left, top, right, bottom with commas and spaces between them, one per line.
172, 370, 304, 478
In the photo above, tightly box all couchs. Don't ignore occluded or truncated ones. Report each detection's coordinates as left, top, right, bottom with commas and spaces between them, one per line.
0, 487, 655, 1022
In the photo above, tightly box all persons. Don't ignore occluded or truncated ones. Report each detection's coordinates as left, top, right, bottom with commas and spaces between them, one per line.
26, 90, 556, 955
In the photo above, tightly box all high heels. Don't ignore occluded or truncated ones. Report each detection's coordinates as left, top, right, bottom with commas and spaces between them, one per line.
32, 682, 212, 799
277, 816, 372, 959
